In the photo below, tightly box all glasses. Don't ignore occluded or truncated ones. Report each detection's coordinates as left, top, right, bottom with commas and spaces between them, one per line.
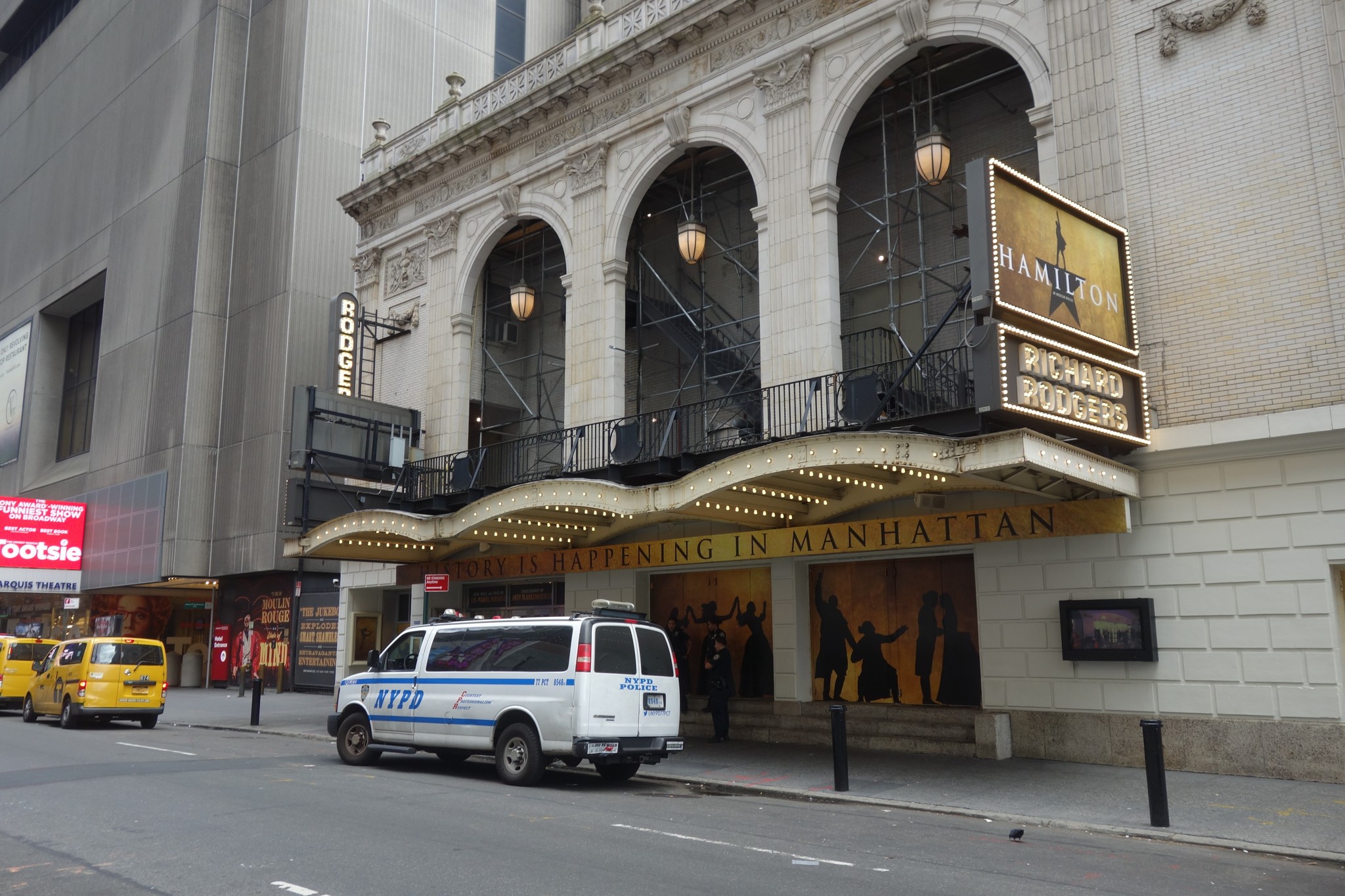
112, 609, 150, 624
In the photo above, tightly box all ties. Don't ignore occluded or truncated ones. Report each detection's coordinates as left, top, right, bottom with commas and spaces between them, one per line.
244, 629, 250, 642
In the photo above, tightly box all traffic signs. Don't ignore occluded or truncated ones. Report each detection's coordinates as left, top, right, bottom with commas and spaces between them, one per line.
424, 574, 449, 592
211, 624, 231, 680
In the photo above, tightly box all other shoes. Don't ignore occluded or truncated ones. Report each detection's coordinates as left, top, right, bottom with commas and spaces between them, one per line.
708, 735, 729, 743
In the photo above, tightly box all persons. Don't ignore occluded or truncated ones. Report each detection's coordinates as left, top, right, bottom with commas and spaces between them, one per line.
664, 617, 733, 741
114, 594, 153, 638
23, 623, 38, 638
231, 610, 261, 687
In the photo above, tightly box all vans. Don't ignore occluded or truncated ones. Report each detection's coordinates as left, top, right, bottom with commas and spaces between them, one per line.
0, 633, 64, 717
328, 598, 685, 789
23, 636, 167, 729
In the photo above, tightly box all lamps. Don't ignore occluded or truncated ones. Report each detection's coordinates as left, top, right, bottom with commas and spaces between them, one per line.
677, 148, 707, 264
509, 219, 536, 321
913, 46, 951, 187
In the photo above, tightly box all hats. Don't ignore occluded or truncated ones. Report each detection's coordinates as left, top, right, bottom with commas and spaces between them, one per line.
242, 609, 252, 621
